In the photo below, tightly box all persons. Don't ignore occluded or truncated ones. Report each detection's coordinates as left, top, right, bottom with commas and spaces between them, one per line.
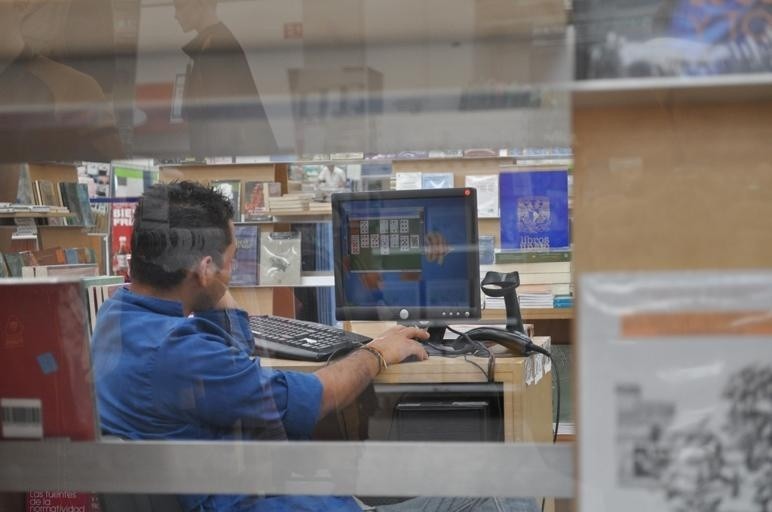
170, 1, 277, 157
86, 180, 542, 511
0, 0, 126, 161
316, 164, 348, 190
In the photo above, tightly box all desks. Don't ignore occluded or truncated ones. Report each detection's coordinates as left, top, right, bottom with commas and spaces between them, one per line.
241, 333, 559, 512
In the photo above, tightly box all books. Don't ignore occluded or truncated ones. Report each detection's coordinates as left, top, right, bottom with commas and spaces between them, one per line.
478, 283, 574, 308
209, 180, 328, 285
0, 161, 158, 330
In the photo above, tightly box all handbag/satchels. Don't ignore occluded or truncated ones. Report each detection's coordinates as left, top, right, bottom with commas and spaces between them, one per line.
359, 345, 387, 376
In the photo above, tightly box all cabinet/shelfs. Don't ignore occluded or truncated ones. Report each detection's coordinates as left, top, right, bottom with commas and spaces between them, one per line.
117, 237, 130, 281
3, 160, 109, 284
158, 156, 580, 322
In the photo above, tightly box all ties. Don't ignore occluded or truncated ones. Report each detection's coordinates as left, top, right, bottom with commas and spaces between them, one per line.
332, 188, 482, 356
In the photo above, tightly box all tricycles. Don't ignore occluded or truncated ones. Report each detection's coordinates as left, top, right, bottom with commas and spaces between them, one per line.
351, 219, 419, 255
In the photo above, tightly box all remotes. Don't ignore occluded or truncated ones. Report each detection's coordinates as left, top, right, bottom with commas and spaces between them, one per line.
403, 349, 429, 362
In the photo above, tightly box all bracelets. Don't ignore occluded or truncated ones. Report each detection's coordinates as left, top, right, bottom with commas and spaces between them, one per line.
359, 345, 387, 376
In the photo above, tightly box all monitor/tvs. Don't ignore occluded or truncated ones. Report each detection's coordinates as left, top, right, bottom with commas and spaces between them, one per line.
478, 283, 574, 308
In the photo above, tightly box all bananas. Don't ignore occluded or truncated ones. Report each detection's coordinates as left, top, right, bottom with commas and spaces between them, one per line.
248, 314, 372, 362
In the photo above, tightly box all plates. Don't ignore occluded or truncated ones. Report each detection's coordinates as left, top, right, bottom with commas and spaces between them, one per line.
3, 160, 109, 284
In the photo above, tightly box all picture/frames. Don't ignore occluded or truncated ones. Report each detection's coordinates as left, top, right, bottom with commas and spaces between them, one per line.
241, 333, 559, 512
